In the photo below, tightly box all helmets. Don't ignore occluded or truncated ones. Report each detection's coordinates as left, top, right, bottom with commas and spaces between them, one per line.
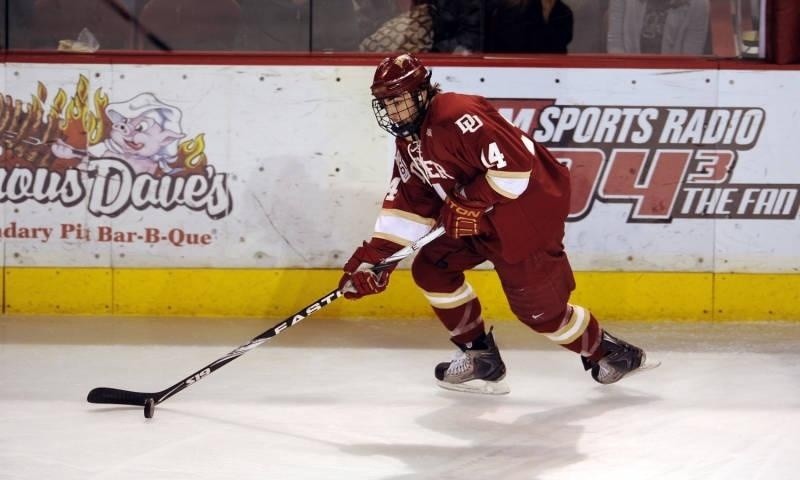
369, 51, 435, 137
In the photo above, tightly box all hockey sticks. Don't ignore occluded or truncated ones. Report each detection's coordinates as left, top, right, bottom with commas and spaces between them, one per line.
88, 205, 492, 407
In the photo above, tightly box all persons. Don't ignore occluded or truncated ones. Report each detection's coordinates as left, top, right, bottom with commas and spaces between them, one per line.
339, 52, 646, 385
460, 0, 574, 55
605, 0, 710, 57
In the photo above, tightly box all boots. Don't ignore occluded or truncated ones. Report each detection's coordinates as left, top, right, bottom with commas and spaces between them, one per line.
581, 330, 644, 385
435, 334, 505, 386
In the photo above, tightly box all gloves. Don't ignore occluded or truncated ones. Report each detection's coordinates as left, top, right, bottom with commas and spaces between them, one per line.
337, 236, 406, 301
440, 193, 491, 240
